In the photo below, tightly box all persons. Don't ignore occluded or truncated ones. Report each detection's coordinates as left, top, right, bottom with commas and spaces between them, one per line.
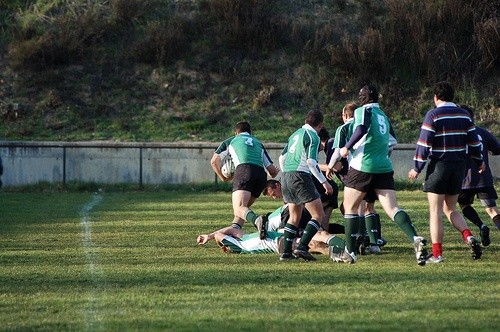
262, 126, 387, 247
279, 109, 333, 261
409, 82, 486, 263
455, 105, 500, 246
210, 121, 280, 239
196, 227, 370, 264
326, 102, 397, 255
331, 84, 428, 266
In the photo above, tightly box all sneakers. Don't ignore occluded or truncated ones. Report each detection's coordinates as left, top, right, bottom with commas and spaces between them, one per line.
364, 245, 381, 253
469, 237, 482, 260
479, 225, 491, 246
376, 237, 387, 247
353, 235, 370, 256
292, 248, 317, 261
255, 215, 268, 240
330, 245, 357, 264
280, 253, 298, 261
412, 236, 428, 266
426, 253, 444, 263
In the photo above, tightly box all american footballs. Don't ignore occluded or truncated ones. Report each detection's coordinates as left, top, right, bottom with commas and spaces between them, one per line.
220, 156, 236, 178
